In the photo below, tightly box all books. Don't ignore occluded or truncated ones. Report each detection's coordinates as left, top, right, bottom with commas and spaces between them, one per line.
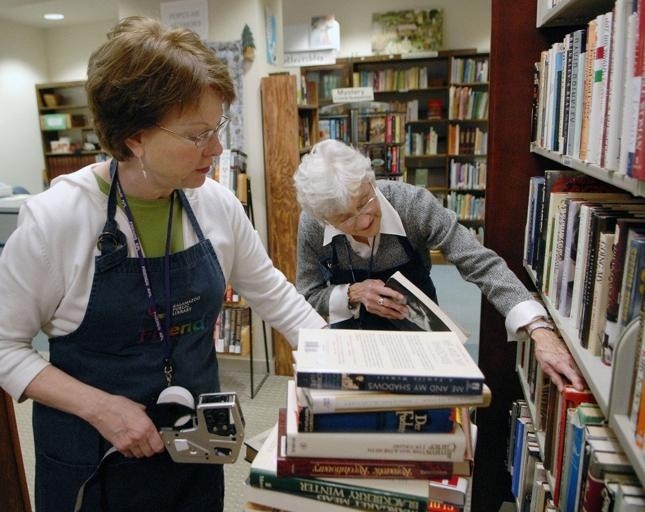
203, 146, 254, 205
212, 286, 492, 512
499, 0, 645, 512
291, 54, 489, 240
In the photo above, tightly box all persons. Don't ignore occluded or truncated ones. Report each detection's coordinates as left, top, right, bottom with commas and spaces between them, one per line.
295, 139, 589, 393
0, 14, 329, 512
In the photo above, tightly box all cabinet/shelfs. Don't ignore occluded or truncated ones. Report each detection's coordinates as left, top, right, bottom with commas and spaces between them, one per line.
30, 2, 642, 511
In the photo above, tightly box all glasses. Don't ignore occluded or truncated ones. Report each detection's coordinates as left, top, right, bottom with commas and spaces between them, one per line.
156, 115, 231, 152
323, 180, 377, 230
